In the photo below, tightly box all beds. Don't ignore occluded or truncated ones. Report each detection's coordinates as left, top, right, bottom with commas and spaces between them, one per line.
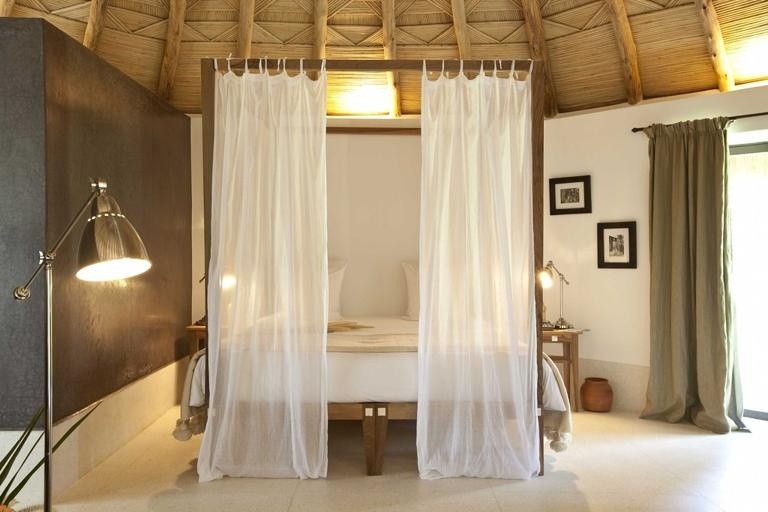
175, 57, 570, 483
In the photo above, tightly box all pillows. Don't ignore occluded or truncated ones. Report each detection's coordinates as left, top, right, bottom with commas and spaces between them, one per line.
265, 260, 351, 323
396, 258, 483, 322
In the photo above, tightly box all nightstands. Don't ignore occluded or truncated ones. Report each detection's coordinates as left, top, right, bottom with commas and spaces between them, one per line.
183, 321, 211, 356
540, 323, 589, 413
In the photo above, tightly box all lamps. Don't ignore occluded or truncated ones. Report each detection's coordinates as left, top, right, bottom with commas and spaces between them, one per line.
13, 174, 154, 511
538, 261, 568, 327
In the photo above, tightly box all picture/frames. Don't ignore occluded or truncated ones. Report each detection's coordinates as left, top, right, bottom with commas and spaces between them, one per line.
548, 174, 591, 217
596, 220, 638, 269
